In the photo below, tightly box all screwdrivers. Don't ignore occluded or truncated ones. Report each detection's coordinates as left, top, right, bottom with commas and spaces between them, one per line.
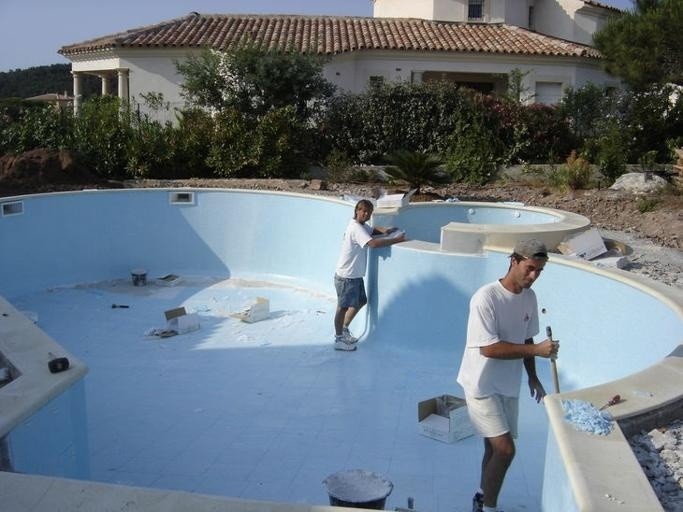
597, 395, 621, 411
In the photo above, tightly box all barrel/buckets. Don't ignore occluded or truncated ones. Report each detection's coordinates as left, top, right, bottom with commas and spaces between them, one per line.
321, 470, 394, 508
131, 269, 148, 286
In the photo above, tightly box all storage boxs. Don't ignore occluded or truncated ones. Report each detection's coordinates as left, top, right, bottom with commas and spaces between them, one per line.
415, 394, 474, 444
156, 272, 180, 286
555, 225, 608, 261
229, 296, 271, 324
375, 187, 418, 209
163, 306, 200, 336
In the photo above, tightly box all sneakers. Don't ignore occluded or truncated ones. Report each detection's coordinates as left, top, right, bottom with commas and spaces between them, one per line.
335, 328, 358, 351
472, 491, 503, 512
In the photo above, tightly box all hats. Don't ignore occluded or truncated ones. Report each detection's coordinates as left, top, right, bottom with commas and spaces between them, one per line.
514, 239, 548, 260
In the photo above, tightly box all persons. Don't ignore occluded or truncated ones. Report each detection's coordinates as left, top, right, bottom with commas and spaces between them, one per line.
455, 237, 559, 511
332, 199, 404, 351
